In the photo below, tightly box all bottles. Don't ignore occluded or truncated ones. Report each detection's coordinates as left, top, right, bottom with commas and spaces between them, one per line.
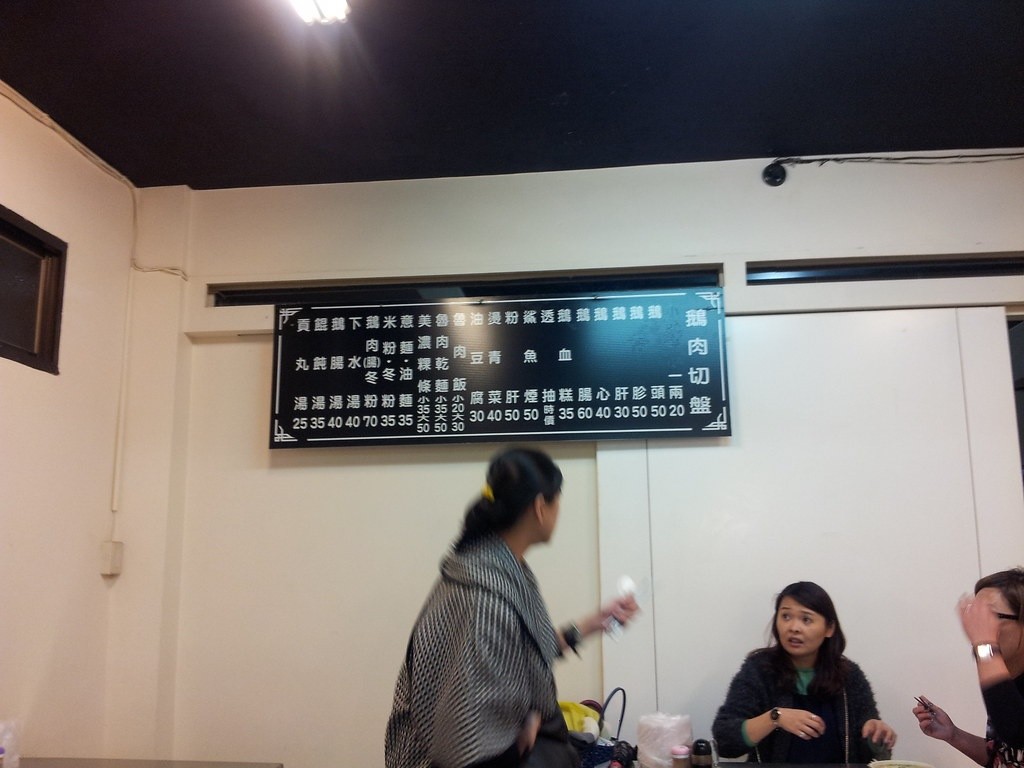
691, 739, 712, 768
672, 745, 689, 768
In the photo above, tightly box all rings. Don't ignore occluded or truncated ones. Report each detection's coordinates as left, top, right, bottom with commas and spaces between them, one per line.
800, 733, 806, 738
812, 715, 817, 720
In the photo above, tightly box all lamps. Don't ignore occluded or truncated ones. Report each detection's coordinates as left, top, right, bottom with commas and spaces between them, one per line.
290, 0, 352, 25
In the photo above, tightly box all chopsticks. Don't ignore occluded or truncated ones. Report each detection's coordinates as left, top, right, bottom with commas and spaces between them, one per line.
914, 697, 934, 714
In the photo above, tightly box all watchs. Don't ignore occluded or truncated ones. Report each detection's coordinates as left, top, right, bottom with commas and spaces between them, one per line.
974, 643, 1000, 660
771, 707, 781, 728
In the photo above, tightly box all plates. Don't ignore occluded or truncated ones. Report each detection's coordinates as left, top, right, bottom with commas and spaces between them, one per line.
867, 760, 934, 768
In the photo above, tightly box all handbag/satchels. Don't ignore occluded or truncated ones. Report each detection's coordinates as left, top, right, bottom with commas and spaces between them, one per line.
569, 687, 637, 768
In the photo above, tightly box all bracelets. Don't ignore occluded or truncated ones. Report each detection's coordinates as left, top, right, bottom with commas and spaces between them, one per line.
564, 624, 579, 648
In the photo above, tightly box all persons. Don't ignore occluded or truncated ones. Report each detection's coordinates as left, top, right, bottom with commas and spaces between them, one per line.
712, 581, 897, 768
913, 571, 1024, 768
386, 447, 636, 768
